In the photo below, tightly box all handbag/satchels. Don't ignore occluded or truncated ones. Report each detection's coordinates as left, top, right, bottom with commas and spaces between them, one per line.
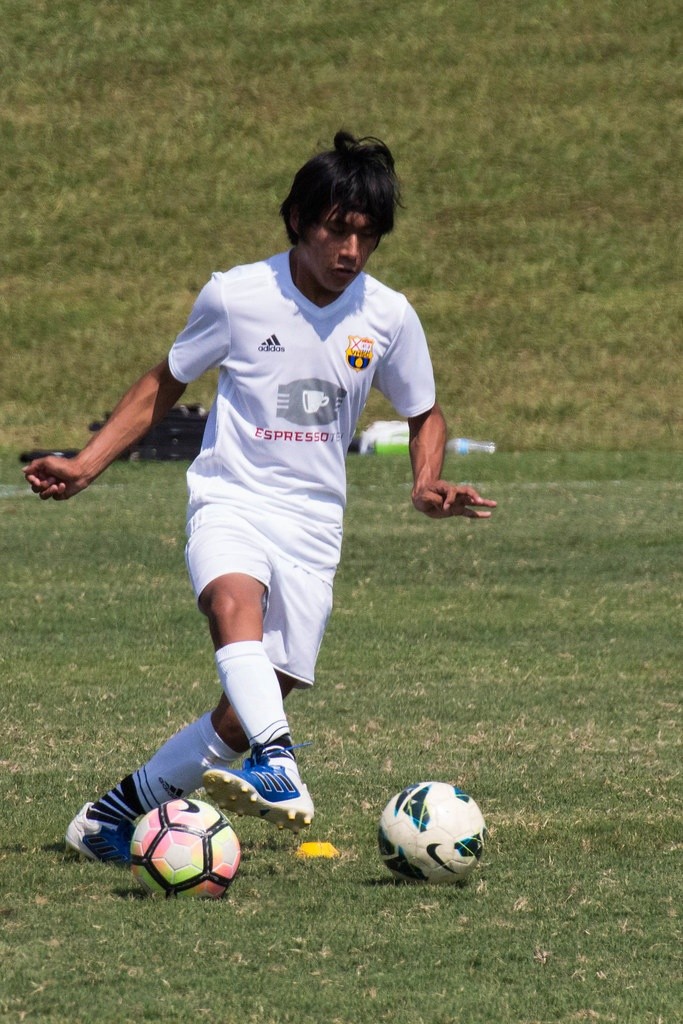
90, 401, 209, 460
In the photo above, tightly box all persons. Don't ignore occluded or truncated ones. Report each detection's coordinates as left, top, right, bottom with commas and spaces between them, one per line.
18, 128, 503, 867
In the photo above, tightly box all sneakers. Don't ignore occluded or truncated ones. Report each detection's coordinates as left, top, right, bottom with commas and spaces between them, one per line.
64, 802, 131, 869
201, 742, 314, 834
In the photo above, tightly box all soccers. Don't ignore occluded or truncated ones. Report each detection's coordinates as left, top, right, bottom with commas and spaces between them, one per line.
377, 782, 485, 884
127, 796, 242, 903
